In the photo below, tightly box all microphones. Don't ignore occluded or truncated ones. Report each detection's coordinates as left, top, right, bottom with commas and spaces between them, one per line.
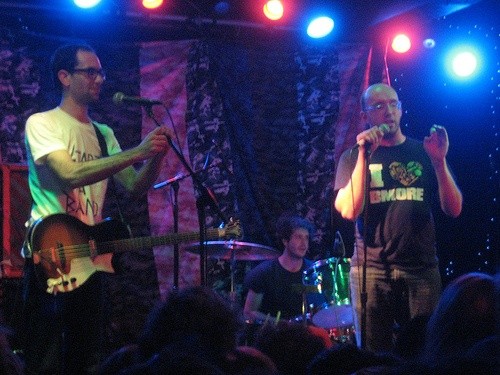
351, 123, 390, 150
333, 233, 341, 257
112, 92, 162, 106
153, 174, 184, 190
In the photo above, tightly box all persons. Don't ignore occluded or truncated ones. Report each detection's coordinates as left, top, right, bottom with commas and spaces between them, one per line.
20, 41, 179, 375
333, 83, 461, 355
0, 216, 500, 375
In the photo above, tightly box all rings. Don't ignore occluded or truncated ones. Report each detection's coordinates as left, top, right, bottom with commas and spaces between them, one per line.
159, 139, 163, 146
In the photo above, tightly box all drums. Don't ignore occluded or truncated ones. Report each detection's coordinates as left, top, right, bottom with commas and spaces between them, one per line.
303, 257, 353, 328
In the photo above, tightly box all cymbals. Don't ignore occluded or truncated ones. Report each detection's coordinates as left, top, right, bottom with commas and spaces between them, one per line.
185, 239, 281, 261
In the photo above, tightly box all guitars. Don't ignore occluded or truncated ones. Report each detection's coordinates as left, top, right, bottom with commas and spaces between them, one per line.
22, 213, 243, 298
